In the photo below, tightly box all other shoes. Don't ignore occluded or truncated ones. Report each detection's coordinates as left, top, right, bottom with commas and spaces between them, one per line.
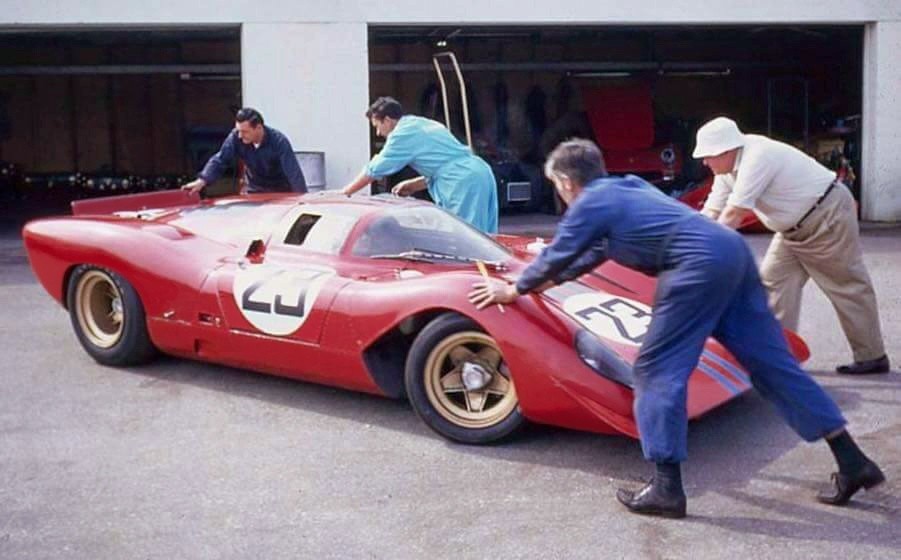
837, 355, 888, 373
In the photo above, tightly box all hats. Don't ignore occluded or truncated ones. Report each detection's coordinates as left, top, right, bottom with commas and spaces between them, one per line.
692, 117, 745, 159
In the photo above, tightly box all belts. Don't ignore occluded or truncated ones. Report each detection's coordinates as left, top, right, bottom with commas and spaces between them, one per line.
785, 177, 838, 233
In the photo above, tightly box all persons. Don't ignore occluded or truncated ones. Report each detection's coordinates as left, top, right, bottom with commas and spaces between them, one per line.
468, 136, 887, 519
691, 117, 890, 375
316, 96, 499, 234
180, 108, 309, 194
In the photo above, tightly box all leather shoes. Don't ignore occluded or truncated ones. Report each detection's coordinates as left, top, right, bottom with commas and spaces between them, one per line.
818, 460, 884, 503
617, 476, 685, 517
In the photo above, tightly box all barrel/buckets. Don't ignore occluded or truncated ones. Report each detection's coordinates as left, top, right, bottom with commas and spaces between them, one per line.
294, 151, 326, 193
